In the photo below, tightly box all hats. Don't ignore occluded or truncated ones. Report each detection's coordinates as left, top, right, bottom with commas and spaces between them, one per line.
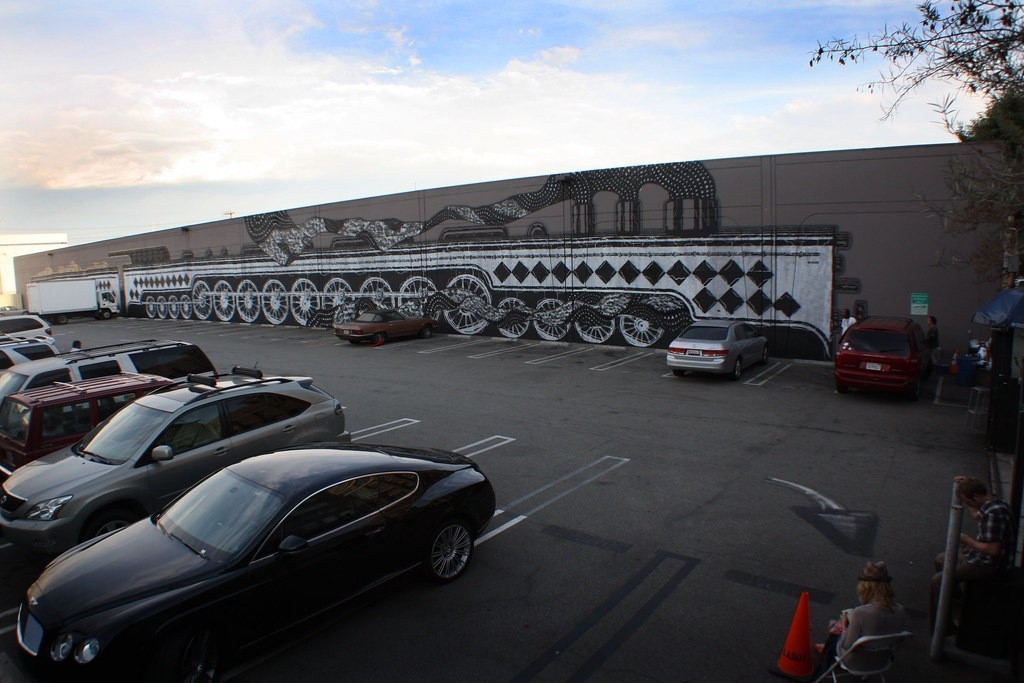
858, 561, 893, 583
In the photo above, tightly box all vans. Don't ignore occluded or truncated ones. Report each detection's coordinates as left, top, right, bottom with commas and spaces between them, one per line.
0, 372, 181, 476
0, 337, 219, 424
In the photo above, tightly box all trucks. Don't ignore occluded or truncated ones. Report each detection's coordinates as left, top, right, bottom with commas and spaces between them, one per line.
27, 276, 121, 325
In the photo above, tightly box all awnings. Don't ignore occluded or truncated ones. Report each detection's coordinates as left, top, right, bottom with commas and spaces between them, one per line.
970, 288, 1024, 329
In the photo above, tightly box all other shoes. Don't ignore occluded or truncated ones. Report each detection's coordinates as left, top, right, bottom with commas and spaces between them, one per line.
813, 643, 829, 663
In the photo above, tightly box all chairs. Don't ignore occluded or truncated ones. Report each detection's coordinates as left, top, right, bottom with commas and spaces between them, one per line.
173, 416, 221, 449
99, 397, 116, 419
812, 632, 914, 683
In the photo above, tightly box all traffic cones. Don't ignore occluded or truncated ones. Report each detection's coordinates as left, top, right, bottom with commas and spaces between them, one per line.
767, 590, 820, 683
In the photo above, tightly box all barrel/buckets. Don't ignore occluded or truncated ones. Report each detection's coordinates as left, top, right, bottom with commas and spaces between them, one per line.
968, 386, 990, 413
956, 356, 978, 387
937, 347, 952, 366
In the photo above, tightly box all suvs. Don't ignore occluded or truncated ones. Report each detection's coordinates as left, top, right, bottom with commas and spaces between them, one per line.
0, 333, 61, 371
0, 364, 353, 555
833, 315, 932, 402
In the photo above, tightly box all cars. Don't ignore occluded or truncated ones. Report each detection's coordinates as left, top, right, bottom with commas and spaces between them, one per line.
0, 305, 53, 338
331, 308, 438, 346
666, 319, 769, 381
10, 440, 497, 683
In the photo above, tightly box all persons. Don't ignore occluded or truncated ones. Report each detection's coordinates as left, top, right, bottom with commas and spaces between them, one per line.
71, 340, 82, 352
927, 477, 1014, 638
922, 316, 942, 371
813, 561, 906, 678
841, 308, 857, 339
